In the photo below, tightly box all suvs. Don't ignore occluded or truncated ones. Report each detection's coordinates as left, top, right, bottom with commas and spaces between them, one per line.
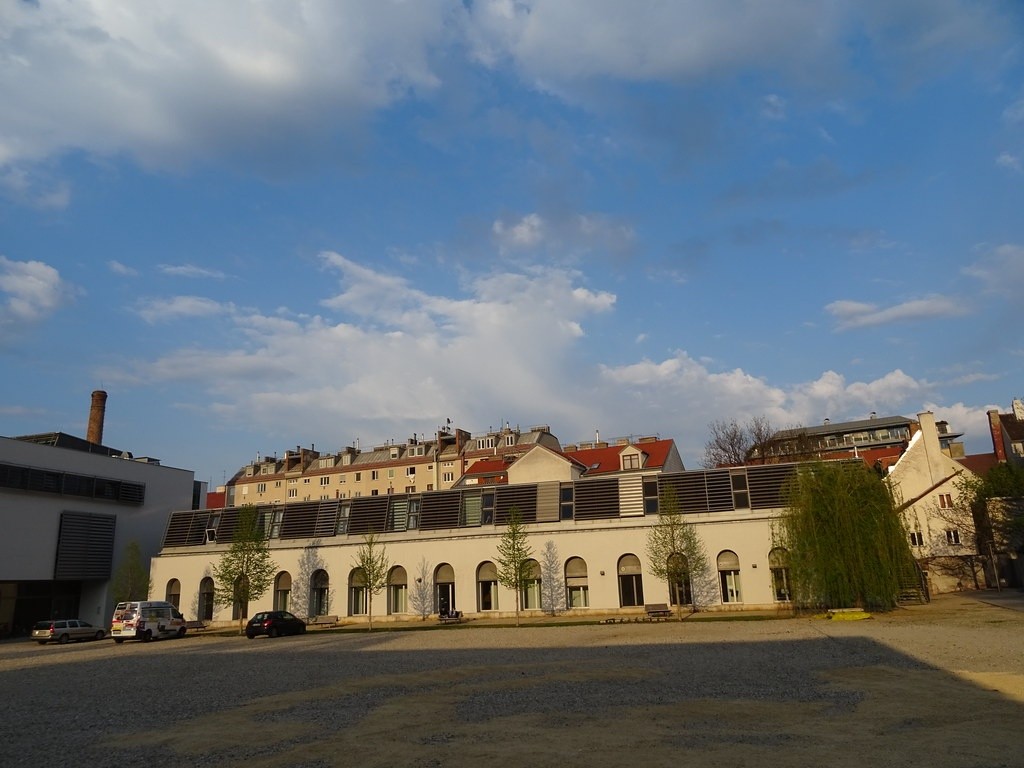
29, 619, 108, 645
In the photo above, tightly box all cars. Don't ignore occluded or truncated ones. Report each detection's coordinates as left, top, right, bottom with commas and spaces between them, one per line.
245, 611, 306, 639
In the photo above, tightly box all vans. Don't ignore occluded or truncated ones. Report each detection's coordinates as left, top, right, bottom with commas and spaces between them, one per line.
110, 600, 188, 644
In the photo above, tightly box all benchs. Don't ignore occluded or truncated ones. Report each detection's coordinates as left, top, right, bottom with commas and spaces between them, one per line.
186, 621, 207, 632
645, 604, 672, 617
313, 616, 338, 628
437, 611, 463, 624
